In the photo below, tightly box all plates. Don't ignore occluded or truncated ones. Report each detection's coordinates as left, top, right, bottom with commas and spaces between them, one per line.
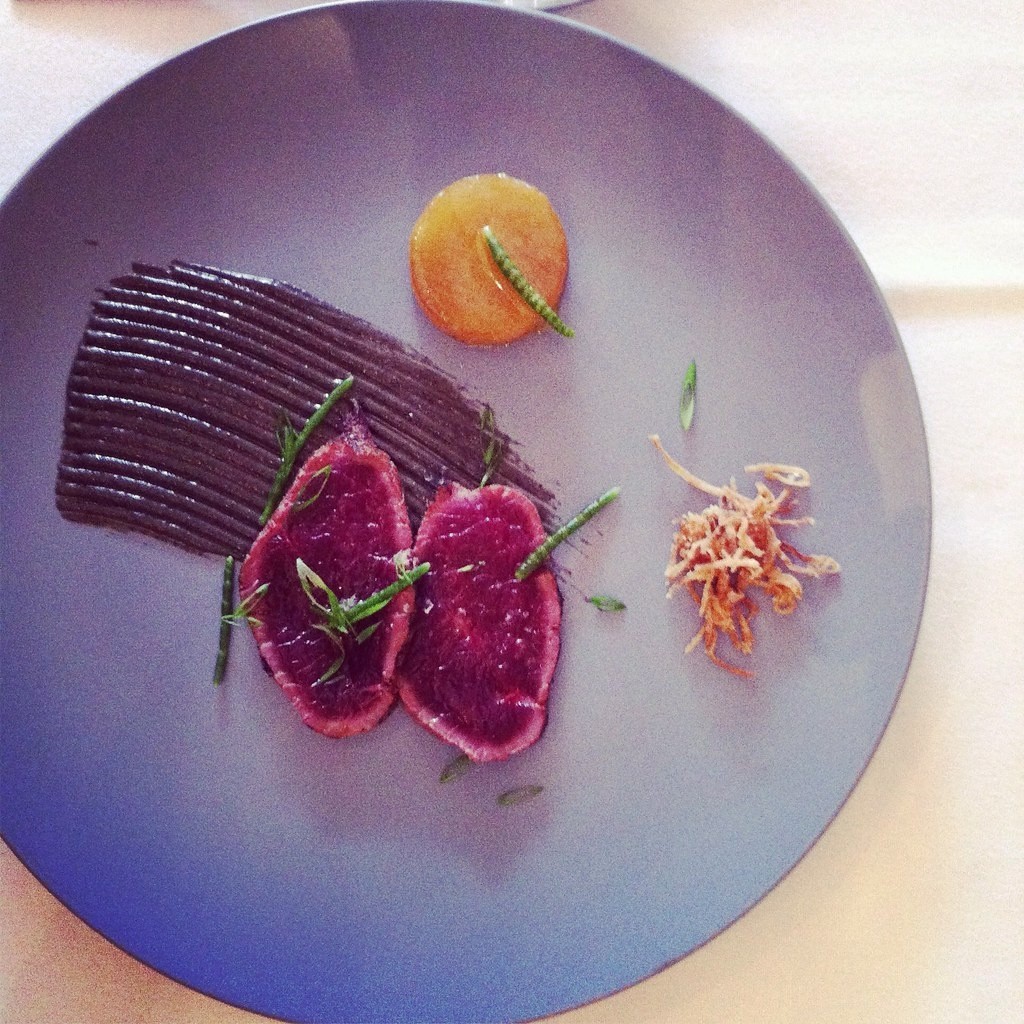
0, 0, 934, 1024
